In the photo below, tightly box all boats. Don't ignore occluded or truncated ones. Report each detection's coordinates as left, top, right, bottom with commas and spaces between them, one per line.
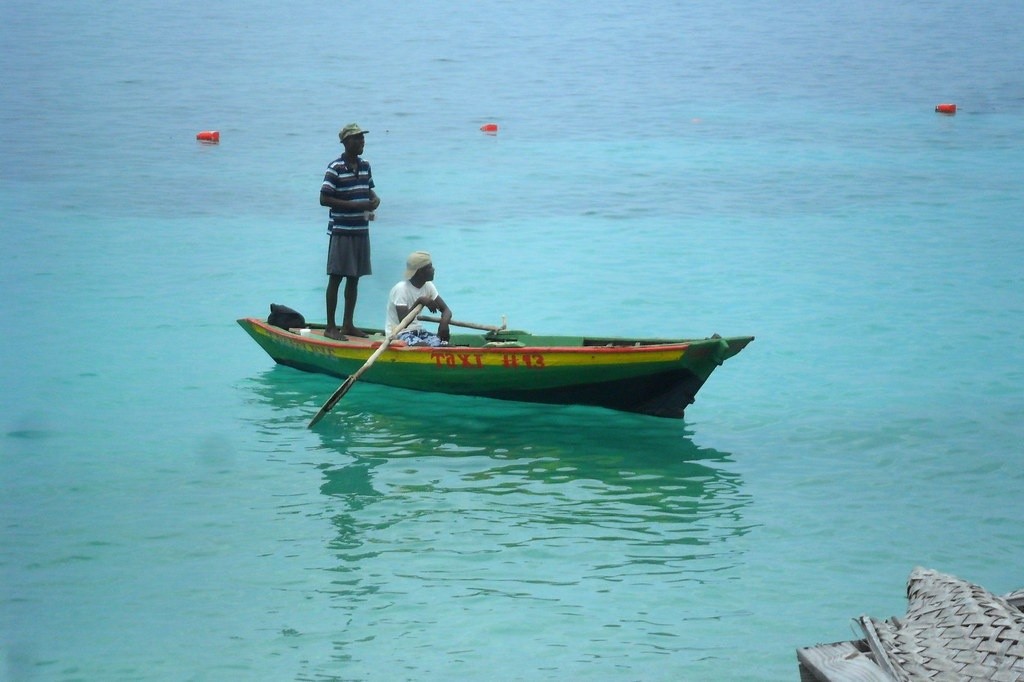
235, 314, 757, 421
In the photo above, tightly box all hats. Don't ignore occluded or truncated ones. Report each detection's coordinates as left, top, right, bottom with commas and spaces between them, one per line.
404, 251, 431, 280
338, 122, 369, 143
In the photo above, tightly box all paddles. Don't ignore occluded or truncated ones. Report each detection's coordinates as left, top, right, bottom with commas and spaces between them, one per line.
416, 313, 501, 332
304, 303, 424, 430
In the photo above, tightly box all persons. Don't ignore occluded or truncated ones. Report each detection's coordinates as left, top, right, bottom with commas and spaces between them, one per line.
385, 253, 452, 347
320, 123, 380, 342
